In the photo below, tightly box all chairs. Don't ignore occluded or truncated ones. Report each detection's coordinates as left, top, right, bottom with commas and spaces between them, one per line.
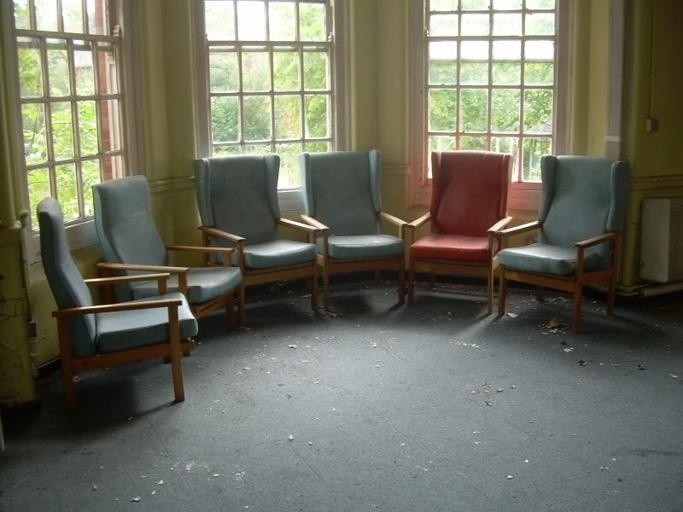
298, 149, 408, 310
33, 195, 198, 421
406, 150, 515, 313
189, 152, 318, 326
90, 174, 243, 347
496, 154, 630, 331
